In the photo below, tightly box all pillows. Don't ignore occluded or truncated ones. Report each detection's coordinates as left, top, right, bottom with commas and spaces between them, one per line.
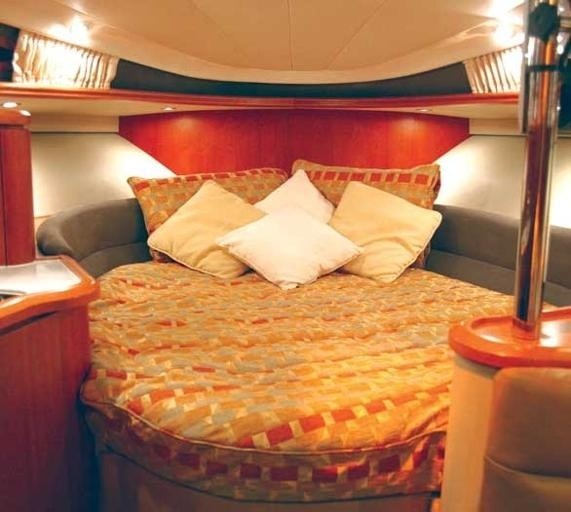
288, 156, 443, 270
250, 168, 336, 226
146, 177, 272, 282
214, 200, 365, 292
126, 166, 291, 266
323, 177, 442, 286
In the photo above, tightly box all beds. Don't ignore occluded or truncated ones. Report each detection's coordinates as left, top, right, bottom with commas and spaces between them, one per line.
29, 188, 570, 512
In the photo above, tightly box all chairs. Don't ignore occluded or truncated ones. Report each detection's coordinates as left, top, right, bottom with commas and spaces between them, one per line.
473, 364, 571, 512
